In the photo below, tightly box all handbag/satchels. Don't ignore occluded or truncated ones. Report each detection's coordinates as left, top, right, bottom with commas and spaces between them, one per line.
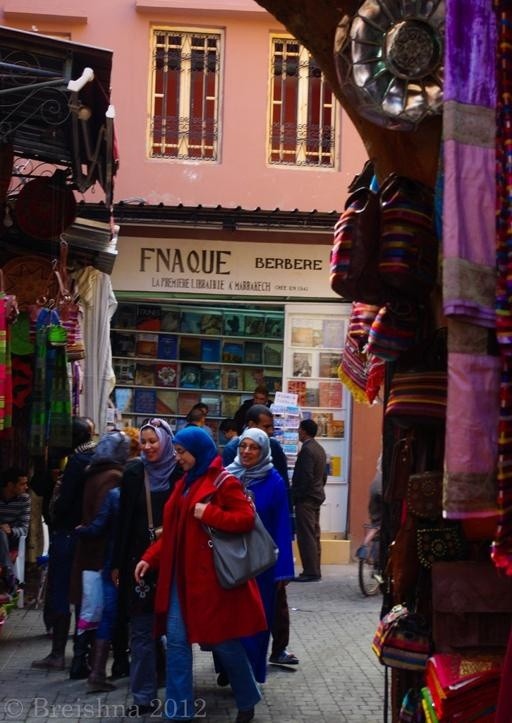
415, 523, 461, 575
406, 470, 442, 518
22, 300, 84, 363
430, 559, 511, 653
207, 499, 280, 590
349, 301, 419, 358
386, 360, 448, 422
372, 603, 429, 676
329, 188, 376, 305
145, 523, 162, 544
378, 172, 438, 309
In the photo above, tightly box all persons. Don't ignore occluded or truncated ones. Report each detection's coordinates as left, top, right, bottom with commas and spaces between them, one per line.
367, 453, 386, 579
1, 387, 328, 722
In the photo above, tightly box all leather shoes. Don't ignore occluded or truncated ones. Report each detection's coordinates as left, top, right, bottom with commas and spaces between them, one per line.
293, 577, 321, 582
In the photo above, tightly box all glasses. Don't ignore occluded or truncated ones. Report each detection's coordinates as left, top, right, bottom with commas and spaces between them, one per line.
175, 449, 185, 455
142, 419, 169, 435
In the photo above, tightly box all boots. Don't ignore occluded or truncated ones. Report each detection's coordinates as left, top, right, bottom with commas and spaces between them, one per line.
33, 613, 71, 667
69, 630, 129, 695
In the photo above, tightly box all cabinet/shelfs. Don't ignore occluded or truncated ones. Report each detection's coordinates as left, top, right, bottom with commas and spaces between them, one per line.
110, 291, 353, 538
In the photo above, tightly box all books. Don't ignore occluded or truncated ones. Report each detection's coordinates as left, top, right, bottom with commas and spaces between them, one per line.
108, 302, 284, 451
267, 316, 349, 480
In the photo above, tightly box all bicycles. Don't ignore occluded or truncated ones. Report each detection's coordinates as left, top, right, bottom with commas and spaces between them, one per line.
357, 524, 385, 595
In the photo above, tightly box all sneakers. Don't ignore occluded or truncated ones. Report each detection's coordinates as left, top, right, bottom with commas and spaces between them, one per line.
235, 706, 255, 723
5, 575, 16, 594
269, 649, 299, 664
127, 705, 156, 715
217, 672, 231, 687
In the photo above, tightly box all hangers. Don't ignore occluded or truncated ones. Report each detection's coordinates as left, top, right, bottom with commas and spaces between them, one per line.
6, 295, 19, 316
35, 257, 72, 306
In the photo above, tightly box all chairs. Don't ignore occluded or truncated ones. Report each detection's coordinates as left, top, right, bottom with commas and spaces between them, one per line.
11, 535, 29, 608
